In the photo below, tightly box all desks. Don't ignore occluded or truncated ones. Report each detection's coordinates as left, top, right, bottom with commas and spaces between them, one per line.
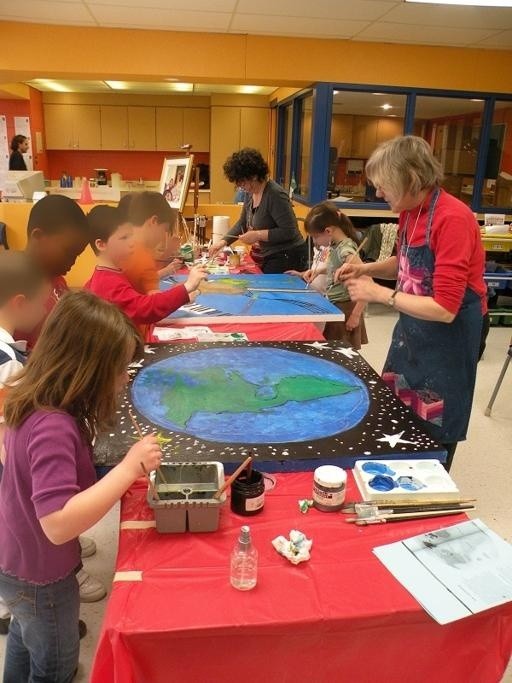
85, 247, 511, 682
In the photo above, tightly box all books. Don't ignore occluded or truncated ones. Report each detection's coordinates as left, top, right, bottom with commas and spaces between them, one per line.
352, 459, 460, 503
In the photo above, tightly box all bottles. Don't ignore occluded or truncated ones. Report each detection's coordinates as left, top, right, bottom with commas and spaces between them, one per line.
60, 175, 96, 188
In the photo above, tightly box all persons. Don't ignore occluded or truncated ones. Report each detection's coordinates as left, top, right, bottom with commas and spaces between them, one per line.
9, 135, 29, 171
0, 251, 88, 641
0, 289, 162, 683
13, 195, 106, 603
83, 190, 211, 345
303, 203, 368, 350
334, 134, 490, 475
209, 147, 309, 274
283, 244, 330, 295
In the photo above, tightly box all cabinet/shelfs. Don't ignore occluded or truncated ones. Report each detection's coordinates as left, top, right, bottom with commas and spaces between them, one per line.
348, 114, 379, 159
206, 93, 269, 203
43, 91, 101, 149
330, 114, 353, 157
152, 94, 211, 153
374, 117, 405, 146
99, 92, 157, 151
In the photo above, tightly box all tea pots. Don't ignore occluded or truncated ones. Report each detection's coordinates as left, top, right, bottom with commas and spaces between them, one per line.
111, 173, 123, 189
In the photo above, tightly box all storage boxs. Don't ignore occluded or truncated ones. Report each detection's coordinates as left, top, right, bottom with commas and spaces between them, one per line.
473, 220, 512, 326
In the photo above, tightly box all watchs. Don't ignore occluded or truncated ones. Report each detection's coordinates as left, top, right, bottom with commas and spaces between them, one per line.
388, 290, 397, 309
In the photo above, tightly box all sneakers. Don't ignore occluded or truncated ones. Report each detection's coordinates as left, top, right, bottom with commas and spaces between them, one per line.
0, 535, 108, 642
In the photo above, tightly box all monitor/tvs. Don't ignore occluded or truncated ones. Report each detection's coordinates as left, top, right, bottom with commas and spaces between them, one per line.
0, 171, 45, 202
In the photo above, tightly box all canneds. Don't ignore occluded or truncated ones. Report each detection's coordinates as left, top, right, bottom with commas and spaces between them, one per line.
313, 465, 347, 512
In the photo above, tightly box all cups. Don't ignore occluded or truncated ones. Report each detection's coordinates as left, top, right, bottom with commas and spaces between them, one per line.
181, 244, 246, 267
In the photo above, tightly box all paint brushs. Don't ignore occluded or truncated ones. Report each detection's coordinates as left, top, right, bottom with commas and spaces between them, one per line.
329, 236, 369, 290
212, 457, 252, 499
140, 462, 161, 501
169, 220, 240, 268
247, 452, 253, 483
304, 253, 323, 290
340, 500, 476, 525
129, 412, 167, 483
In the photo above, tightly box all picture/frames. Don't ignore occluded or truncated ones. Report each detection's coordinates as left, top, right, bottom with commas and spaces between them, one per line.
157, 153, 195, 212
